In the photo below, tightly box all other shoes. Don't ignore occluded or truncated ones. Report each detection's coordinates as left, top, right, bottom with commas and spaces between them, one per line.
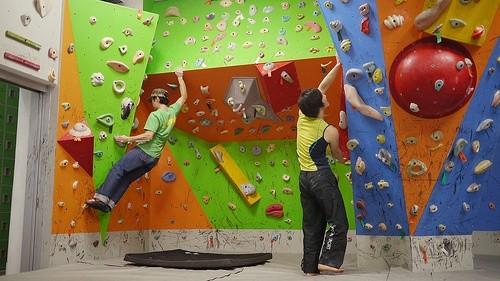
100, 204, 111, 213
85, 198, 106, 209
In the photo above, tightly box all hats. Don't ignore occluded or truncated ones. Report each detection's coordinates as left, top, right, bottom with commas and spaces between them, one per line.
146, 88, 169, 103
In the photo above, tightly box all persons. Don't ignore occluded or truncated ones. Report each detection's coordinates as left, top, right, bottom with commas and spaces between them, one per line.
85, 67, 188, 213
296, 52, 349, 276
